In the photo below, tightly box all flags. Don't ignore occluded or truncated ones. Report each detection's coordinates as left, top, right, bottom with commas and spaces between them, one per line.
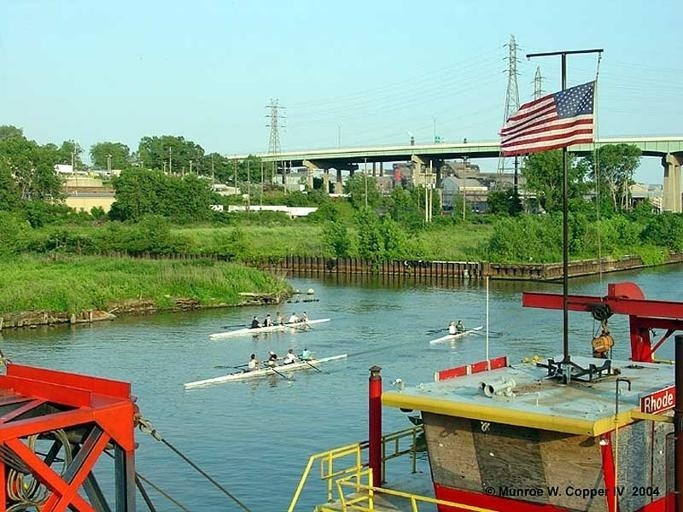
498, 78, 595, 157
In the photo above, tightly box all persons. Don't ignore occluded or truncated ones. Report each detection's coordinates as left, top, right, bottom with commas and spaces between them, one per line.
448, 321, 457, 335
263, 314, 270, 327
456, 320, 465, 334
247, 353, 259, 371
288, 312, 298, 323
282, 348, 297, 364
274, 311, 283, 325
300, 311, 306, 322
267, 350, 277, 367
249, 315, 260, 328
298, 347, 313, 363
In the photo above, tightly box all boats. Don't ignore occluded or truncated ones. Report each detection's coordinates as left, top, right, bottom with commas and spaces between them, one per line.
182, 353, 348, 389
429, 326, 483, 346
209, 318, 330, 341
